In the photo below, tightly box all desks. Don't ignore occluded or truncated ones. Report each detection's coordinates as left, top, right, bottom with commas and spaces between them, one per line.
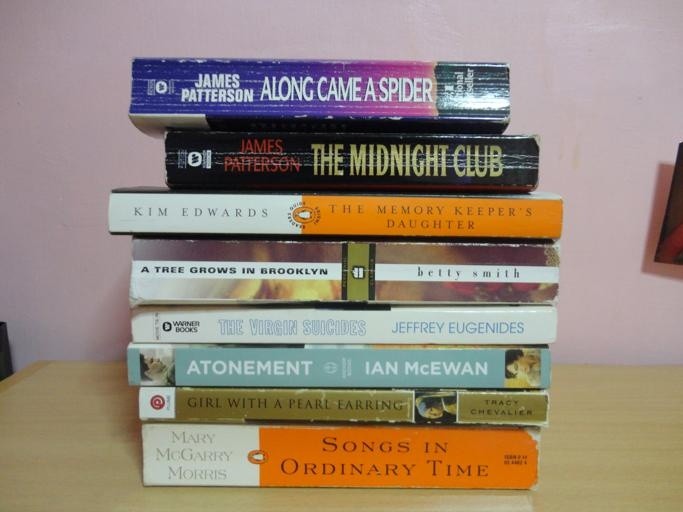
0, 360, 682, 512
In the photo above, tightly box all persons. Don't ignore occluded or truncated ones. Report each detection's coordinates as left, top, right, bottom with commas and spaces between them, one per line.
505, 350, 541, 385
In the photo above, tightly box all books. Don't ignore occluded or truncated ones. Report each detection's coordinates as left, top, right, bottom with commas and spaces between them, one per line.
131, 304, 556, 345
142, 422, 541, 490
128, 342, 551, 391
138, 386, 550, 427
130, 237, 561, 307
163, 128, 539, 192
130, 58, 511, 133
109, 186, 564, 244
